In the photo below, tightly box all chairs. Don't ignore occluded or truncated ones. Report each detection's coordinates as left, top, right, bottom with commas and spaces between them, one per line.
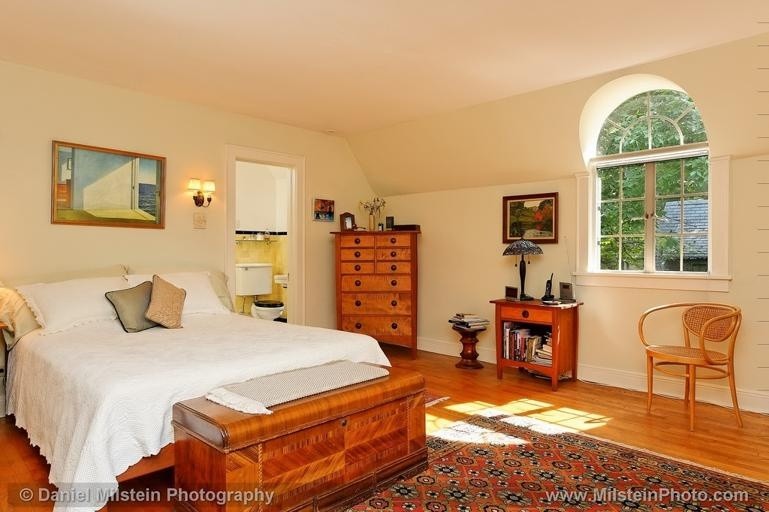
638, 301, 745, 434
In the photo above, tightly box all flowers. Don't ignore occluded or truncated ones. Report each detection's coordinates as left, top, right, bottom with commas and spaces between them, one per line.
359, 191, 387, 219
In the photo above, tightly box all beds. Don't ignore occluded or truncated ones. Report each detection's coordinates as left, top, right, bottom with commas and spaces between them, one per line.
1, 270, 392, 512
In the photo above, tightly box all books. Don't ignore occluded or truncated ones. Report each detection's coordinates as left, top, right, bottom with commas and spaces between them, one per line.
559, 282, 572, 299
448, 312, 491, 330
502, 321, 552, 368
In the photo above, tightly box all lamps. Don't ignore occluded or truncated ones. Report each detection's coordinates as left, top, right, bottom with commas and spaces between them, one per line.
501, 238, 544, 301
185, 176, 218, 208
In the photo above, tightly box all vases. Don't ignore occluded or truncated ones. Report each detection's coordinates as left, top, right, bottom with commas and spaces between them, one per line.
369, 215, 376, 231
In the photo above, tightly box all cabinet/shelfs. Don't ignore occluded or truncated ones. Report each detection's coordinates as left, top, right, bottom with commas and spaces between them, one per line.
330, 230, 421, 362
451, 325, 488, 370
487, 299, 585, 394
57, 182, 70, 209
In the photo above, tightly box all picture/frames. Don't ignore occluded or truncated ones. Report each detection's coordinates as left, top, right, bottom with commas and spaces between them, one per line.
50, 139, 167, 230
502, 192, 559, 245
67, 157, 72, 170
339, 210, 355, 232
311, 196, 336, 223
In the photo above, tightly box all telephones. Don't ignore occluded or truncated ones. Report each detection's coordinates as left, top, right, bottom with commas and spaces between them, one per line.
542, 273, 554, 301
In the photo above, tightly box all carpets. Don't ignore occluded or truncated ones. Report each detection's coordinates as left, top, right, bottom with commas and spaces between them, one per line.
331, 407, 767, 512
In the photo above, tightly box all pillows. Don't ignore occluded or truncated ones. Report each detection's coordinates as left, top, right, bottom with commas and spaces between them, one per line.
104, 281, 160, 333
123, 269, 232, 317
1, 287, 41, 352
143, 274, 187, 329
207, 271, 233, 312
11, 272, 131, 337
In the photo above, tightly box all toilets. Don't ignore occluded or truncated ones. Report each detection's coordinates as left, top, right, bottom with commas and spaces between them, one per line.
235, 263, 284, 320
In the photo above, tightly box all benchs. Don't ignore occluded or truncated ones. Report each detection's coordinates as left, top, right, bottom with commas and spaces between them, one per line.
172, 361, 426, 512
